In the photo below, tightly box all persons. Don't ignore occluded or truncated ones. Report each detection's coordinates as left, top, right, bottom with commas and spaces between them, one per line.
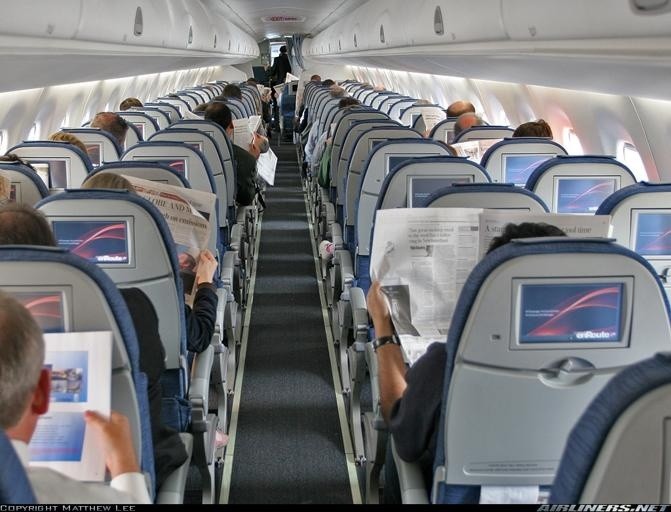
1, 153, 37, 173
364, 219, 569, 460
1, 289, 154, 504
512, 118, 554, 140
0, 200, 188, 489
416, 99, 432, 104
47, 132, 89, 157
80, 171, 219, 355
193, 77, 273, 205
119, 97, 143, 113
271, 46, 291, 84
445, 100, 476, 118
292, 73, 386, 189
453, 112, 483, 136
90, 111, 130, 149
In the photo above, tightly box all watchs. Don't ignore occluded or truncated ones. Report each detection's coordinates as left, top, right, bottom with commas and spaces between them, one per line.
370, 335, 401, 353
